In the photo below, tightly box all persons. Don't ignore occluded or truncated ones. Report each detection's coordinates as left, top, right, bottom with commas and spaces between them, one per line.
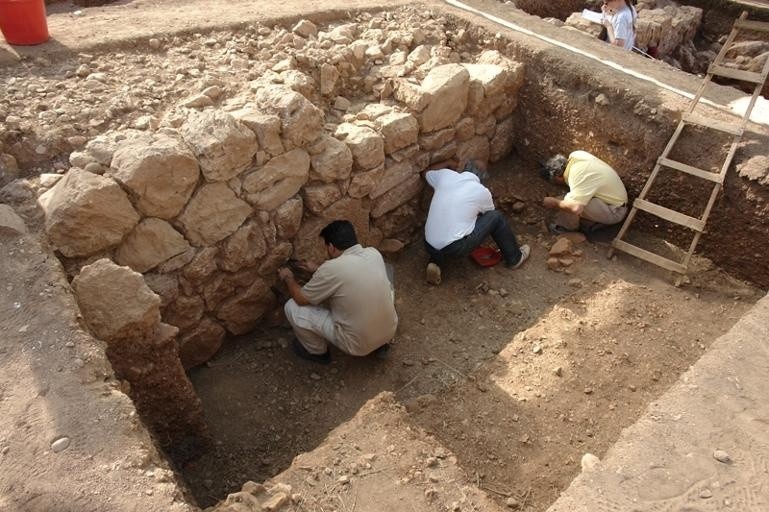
420, 158, 531, 285
601, 0, 639, 51
542, 150, 629, 236
277, 220, 399, 365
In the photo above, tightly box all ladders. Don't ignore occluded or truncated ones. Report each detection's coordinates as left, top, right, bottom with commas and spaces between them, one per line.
606, 11, 769, 288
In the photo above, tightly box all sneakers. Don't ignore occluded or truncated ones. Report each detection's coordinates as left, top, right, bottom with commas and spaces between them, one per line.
425, 263, 442, 287
548, 223, 571, 237
291, 337, 331, 365
508, 243, 532, 270
377, 342, 391, 361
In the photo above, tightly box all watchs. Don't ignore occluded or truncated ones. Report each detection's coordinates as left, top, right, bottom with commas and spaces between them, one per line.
284, 272, 295, 283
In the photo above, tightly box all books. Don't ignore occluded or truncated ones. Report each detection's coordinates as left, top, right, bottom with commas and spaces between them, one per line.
581, 9, 611, 25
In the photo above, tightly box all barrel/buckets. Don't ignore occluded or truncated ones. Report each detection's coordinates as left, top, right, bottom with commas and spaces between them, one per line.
0, 0, 49, 46
